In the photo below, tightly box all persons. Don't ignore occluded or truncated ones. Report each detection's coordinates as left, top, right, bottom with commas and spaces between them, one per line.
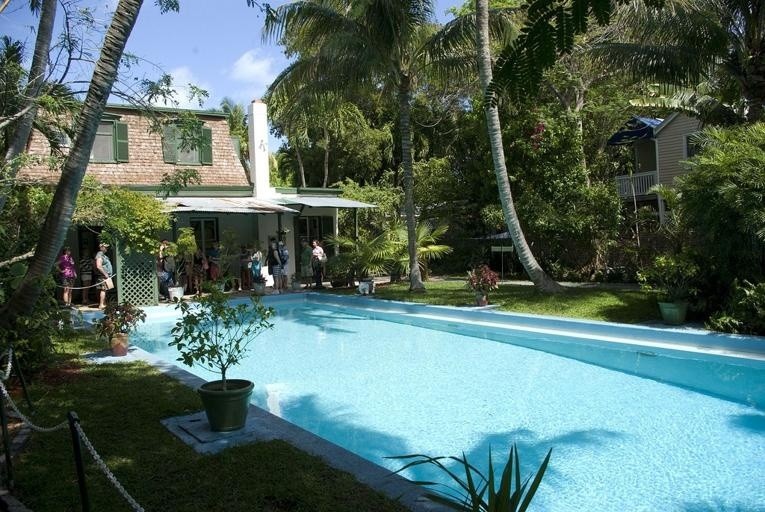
311, 239, 328, 289
53, 247, 74, 307
298, 239, 315, 288
94, 242, 114, 309
76, 246, 96, 306
157, 240, 264, 301
268, 235, 282, 294
278, 238, 290, 290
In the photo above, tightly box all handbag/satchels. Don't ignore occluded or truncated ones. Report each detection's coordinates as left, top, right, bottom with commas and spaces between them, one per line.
104, 279, 114, 290
317, 253, 328, 263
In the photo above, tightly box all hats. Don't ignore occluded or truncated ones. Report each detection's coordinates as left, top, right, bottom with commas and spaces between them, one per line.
99, 242, 110, 247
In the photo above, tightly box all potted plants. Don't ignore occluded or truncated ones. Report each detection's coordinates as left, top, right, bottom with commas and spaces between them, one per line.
90, 294, 149, 356
462, 263, 500, 307
636, 252, 704, 326
160, 231, 200, 304
166, 275, 279, 432
321, 229, 396, 295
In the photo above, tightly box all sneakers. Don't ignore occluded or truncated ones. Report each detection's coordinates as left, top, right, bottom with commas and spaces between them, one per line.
271, 285, 288, 295
230, 287, 255, 293
195, 289, 201, 296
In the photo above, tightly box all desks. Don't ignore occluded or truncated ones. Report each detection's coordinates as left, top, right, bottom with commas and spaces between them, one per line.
209, 259, 253, 291
